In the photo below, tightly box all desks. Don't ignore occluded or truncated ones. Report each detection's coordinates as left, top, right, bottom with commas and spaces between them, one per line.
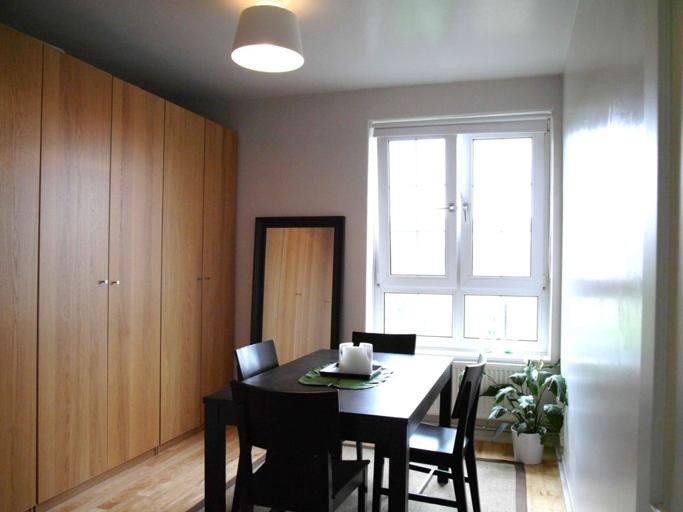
202, 348, 453, 508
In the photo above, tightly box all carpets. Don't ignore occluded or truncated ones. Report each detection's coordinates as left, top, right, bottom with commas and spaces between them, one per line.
189, 443, 529, 511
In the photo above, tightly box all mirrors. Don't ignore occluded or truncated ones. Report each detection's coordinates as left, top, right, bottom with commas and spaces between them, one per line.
248, 214, 347, 367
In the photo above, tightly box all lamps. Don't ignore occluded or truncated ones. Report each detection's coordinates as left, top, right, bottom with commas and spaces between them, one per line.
226, 4, 305, 77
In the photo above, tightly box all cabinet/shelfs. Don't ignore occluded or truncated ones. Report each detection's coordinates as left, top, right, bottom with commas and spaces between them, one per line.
34, 41, 164, 511
1, 30, 42, 511
155, 100, 239, 454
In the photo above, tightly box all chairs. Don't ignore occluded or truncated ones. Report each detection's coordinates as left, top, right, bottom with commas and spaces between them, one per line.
228, 330, 487, 512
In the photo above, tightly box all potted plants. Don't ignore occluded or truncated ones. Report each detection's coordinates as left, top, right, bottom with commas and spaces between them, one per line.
476, 358, 567, 464
